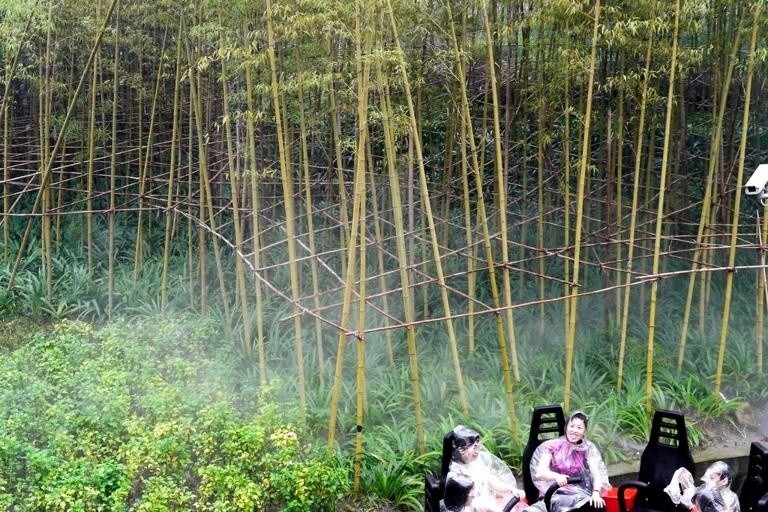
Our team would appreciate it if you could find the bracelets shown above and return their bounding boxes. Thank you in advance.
[592,490,600,493]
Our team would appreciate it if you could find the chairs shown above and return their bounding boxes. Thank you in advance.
[427,428,521,512]
[523,406,566,506]
[630,445,693,512]
[637,406,699,485]
[736,440,766,511]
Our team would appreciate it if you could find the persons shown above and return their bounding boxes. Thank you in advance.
[676,459,740,512]
[527,411,613,511]
[443,474,477,512]
[444,426,531,512]
[689,489,728,512]
